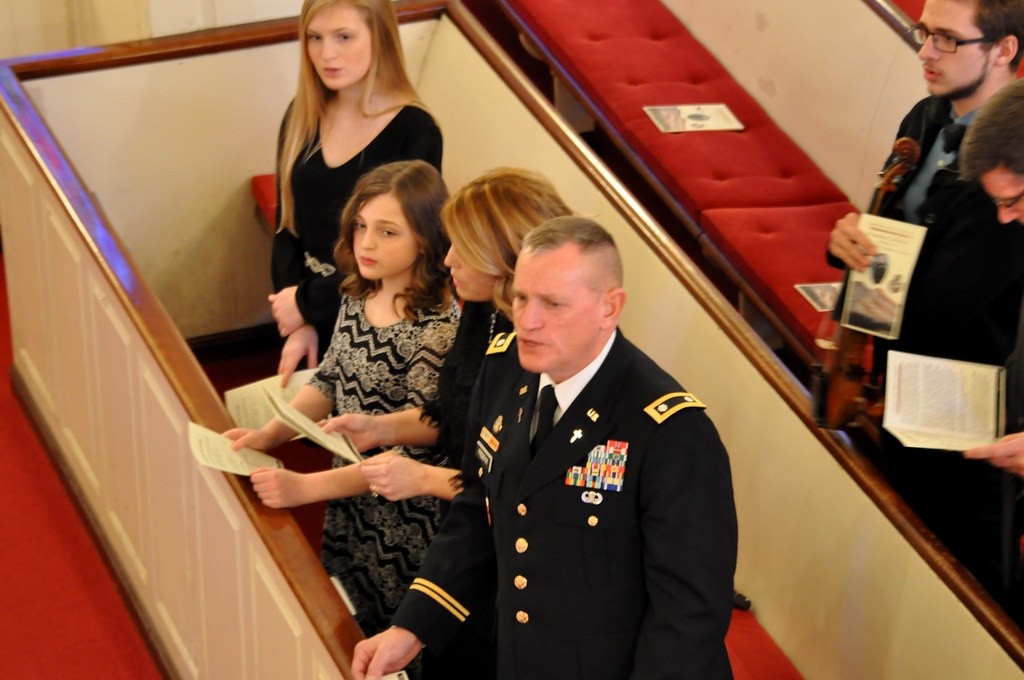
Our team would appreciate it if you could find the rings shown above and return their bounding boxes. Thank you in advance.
[371,485,378,498]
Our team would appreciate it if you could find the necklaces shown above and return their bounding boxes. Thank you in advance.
[488,307,499,344]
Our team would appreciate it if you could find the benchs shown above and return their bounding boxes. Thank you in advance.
[496,0,918,373]
[252,173,805,680]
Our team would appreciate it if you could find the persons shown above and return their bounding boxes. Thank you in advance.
[824,0,1024,610]
[219,1,739,680]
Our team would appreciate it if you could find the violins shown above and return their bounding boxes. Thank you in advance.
[811,135,923,432]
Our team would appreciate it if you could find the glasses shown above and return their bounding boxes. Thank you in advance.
[992,188,1024,209]
[911,23,1004,53]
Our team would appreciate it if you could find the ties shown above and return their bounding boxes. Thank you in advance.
[530,384,556,453]
